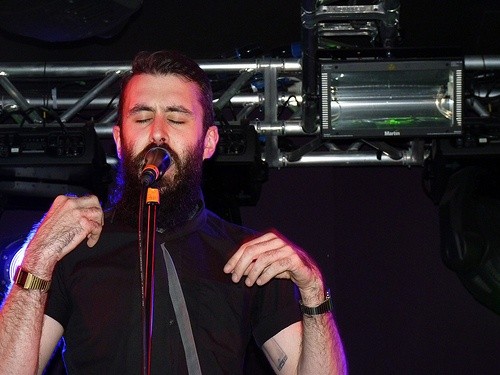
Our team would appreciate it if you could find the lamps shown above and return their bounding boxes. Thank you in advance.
[300,0,463,140]
[201,123,269,207]
[0,109,113,211]
[423,117,500,315]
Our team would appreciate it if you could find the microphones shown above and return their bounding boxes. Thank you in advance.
[139,146,172,186]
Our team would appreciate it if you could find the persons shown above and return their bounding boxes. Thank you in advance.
[0,49,347,375]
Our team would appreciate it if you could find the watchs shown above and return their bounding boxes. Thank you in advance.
[12,267,52,292]
[298,288,336,315]
